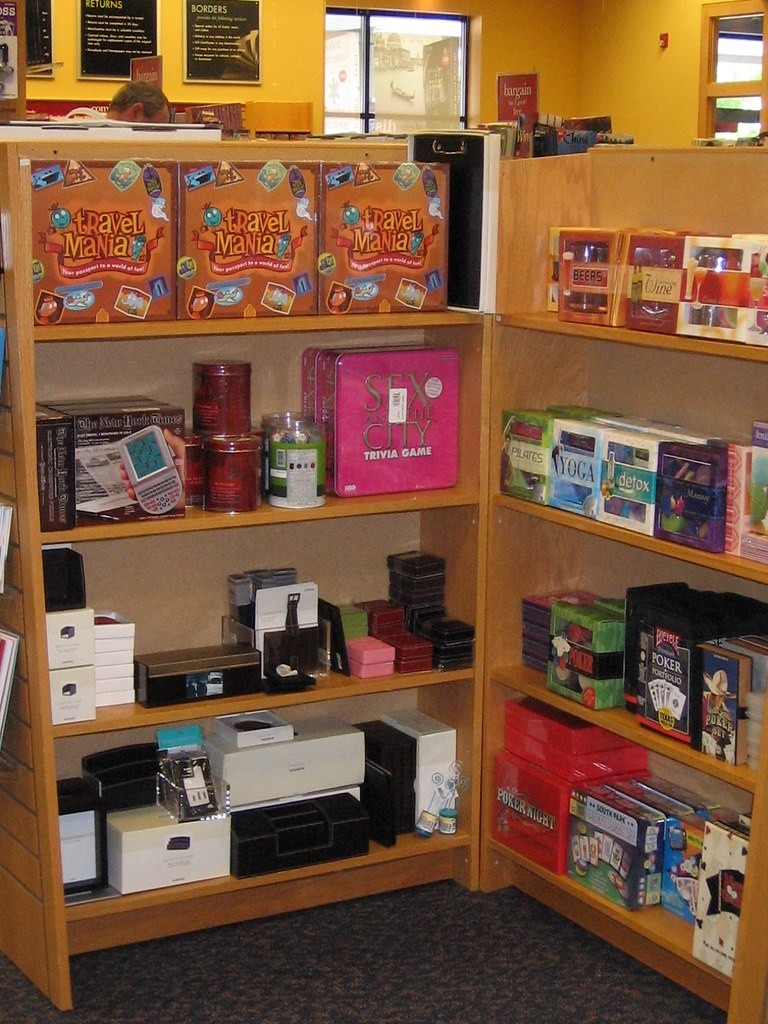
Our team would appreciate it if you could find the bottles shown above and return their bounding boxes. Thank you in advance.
[178,360,331,515]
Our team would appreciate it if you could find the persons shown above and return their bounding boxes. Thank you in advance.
[103,78,172,131]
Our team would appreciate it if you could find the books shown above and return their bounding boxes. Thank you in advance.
[476,109,768,161]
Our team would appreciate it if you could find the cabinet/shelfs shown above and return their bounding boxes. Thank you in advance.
[479,149,768,1024]
[1,140,490,1012]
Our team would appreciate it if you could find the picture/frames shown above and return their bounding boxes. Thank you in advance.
[76,0,160,81]
[182,0,261,85]
[25,0,53,78]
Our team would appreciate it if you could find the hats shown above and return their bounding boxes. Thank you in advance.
[702,670,736,700]
